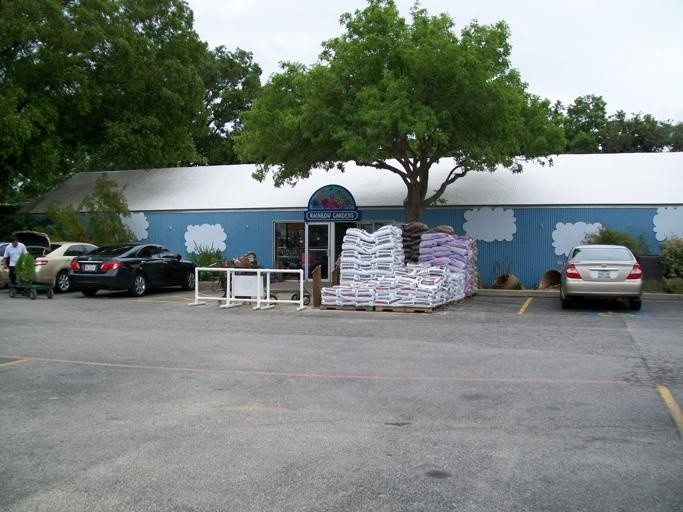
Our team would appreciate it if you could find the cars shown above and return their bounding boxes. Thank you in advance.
[0,230,99,291]
[557,245,644,311]
[68,244,200,297]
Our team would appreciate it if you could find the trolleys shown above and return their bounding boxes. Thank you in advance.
[8,284,54,299]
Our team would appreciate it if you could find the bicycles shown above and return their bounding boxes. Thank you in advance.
[291,286,311,305]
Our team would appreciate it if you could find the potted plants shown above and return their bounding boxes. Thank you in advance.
[13,252,36,286]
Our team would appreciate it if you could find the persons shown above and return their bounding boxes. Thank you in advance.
[3,236,29,294]
